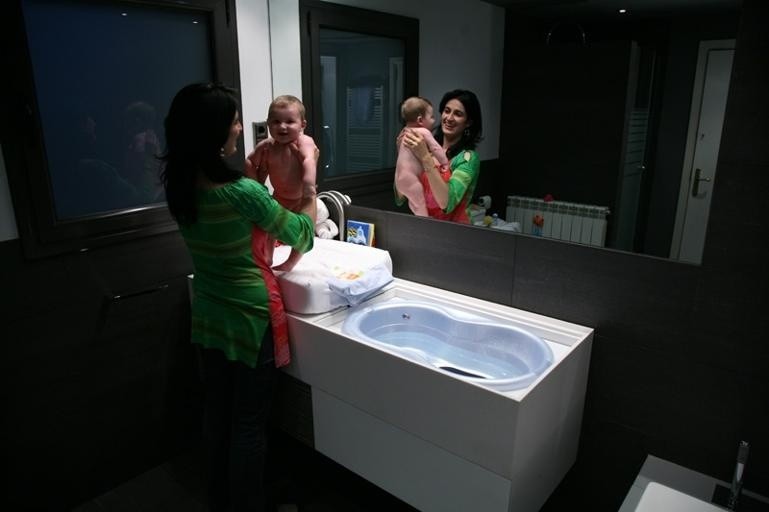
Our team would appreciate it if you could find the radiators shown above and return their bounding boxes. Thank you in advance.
[505,195,611,249]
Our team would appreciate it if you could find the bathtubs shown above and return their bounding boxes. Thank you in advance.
[343,295,554,389]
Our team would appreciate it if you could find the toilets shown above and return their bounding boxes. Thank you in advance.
[634,480,727,512]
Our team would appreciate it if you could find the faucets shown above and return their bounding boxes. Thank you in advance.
[318,190,347,239]
[725,441,748,507]
[329,188,350,208]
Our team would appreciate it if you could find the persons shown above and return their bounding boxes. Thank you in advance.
[247,96,318,272]
[392,88,485,228]
[65,96,162,212]
[122,101,163,183]
[152,81,320,512]
[395,96,450,218]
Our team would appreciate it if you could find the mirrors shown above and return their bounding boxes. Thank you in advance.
[297,0,744,269]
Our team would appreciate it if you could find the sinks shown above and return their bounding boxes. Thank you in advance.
[268,236,393,314]
